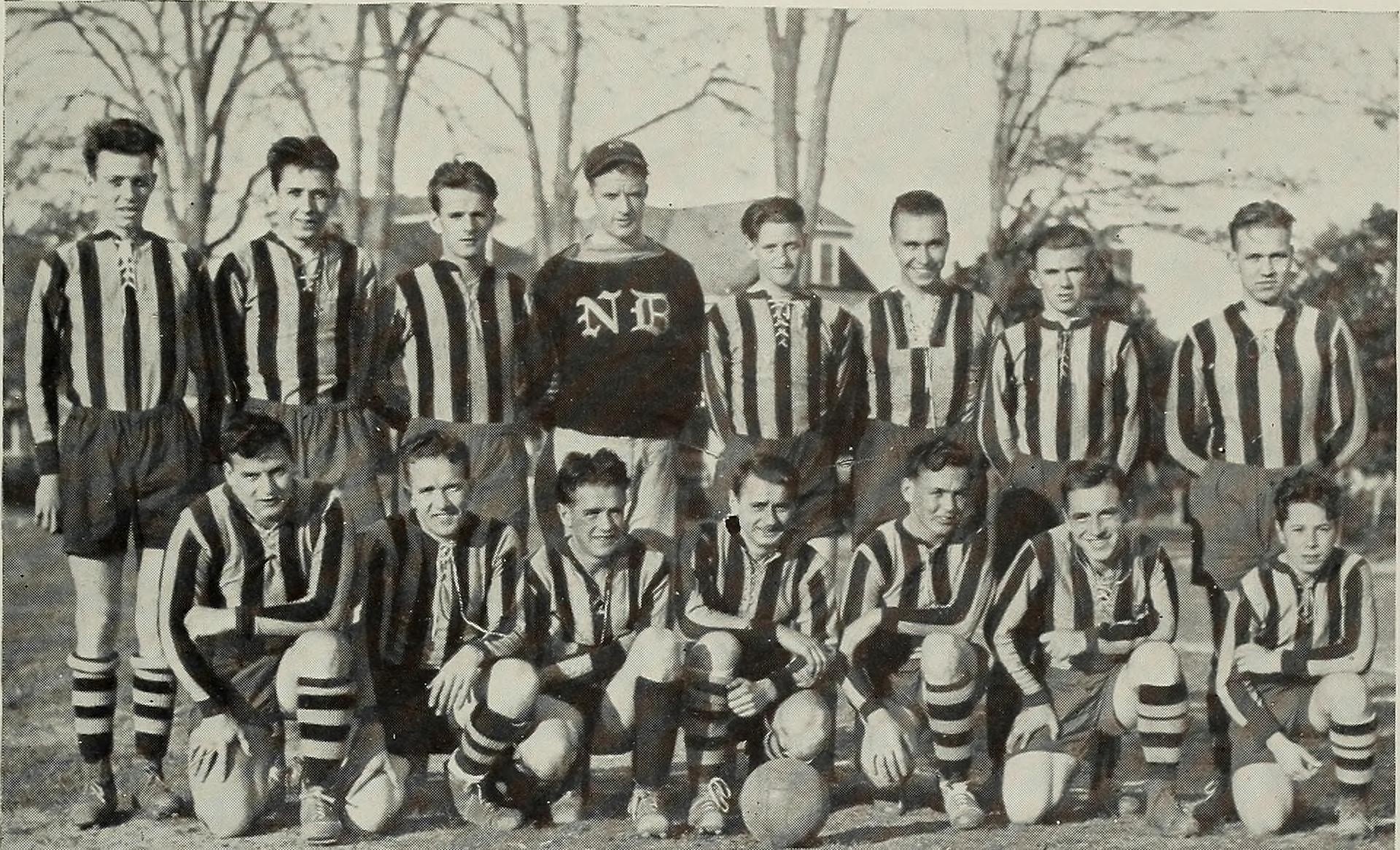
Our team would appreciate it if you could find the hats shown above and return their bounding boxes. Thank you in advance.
[584,138,649,183]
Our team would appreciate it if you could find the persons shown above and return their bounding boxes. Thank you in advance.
[153,410,364,849]
[836,434,1001,831]
[365,156,549,552]
[974,221,1148,819]
[344,427,542,835]
[669,448,844,835]
[18,117,235,828]
[523,136,708,630]
[848,190,1006,557]
[212,135,387,609]
[1159,196,1371,824]
[981,457,1204,838]
[697,194,864,582]
[507,447,691,840]
[1212,464,1378,840]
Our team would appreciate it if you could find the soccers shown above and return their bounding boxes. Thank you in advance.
[739,756,831,848]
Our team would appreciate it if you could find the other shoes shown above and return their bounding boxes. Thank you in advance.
[1088,733,1119,815]
[1192,777,1238,824]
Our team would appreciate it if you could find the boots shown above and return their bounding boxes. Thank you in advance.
[441,760,523,832]
[1145,777,1201,838]
[71,754,119,828]
[130,754,186,821]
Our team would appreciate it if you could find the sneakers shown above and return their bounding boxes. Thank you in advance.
[299,775,342,845]
[688,778,727,835]
[267,721,287,807]
[534,741,593,825]
[873,788,907,817]
[629,782,671,838]
[936,770,985,829]
[1337,786,1373,838]
